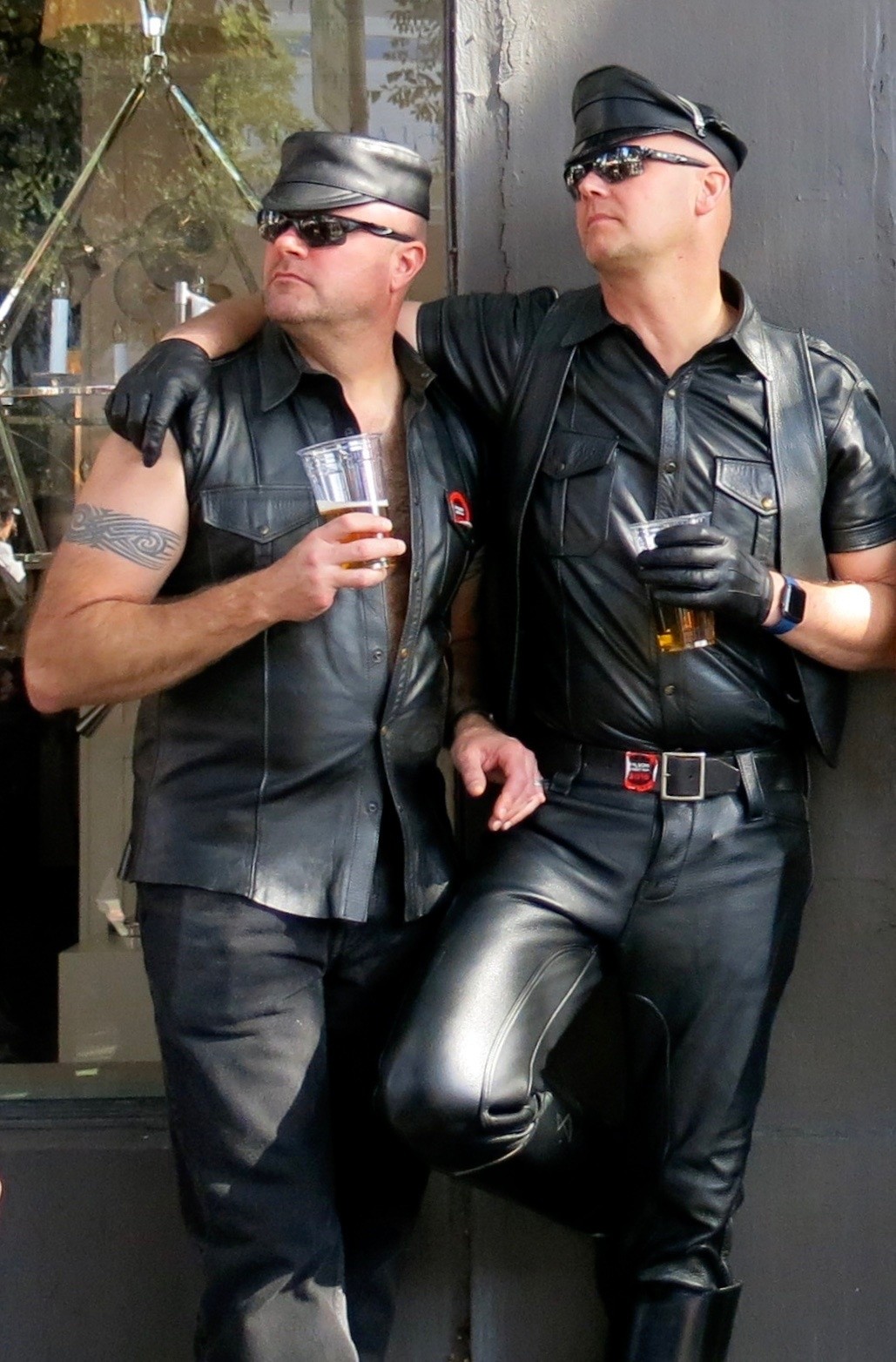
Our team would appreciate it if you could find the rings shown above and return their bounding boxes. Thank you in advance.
[534,782,543,787]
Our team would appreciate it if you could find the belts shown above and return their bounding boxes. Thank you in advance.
[516,699,804,800]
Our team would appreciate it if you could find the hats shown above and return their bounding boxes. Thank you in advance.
[260,131,434,221]
[565,65,749,188]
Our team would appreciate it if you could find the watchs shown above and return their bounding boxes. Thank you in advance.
[762,572,807,634]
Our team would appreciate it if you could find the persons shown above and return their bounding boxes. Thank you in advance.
[0,490,27,686]
[21,129,547,1362]
[103,66,896,1362]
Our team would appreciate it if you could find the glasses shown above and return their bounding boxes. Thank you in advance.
[563,147,711,201]
[257,205,415,248]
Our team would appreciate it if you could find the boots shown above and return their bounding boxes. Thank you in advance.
[450,1089,623,1305]
[606,1279,744,1362]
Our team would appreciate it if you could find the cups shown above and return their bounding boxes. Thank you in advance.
[296,434,397,578]
[629,512,718,653]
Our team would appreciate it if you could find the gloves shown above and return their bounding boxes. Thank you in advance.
[634,525,773,627]
[104,338,215,468]
